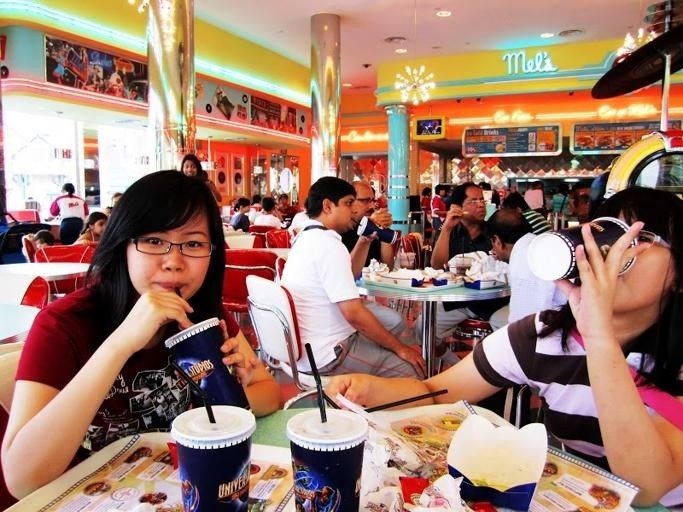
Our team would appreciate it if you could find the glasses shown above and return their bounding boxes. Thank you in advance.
[354,197,378,204]
[633,228,675,253]
[126,236,218,259]
[463,198,489,207]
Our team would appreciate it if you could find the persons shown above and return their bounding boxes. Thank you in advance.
[50,49,67,84]
[428,120,440,134]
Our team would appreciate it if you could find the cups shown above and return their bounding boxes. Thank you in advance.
[357,216,398,245]
[528,216,639,281]
[171,405,257,512]
[284,408,368,512]
[164,318,253,414]
[455,256,473,277]
[400,253,416,268]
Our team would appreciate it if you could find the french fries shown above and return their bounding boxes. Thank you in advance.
[468,475,511,493]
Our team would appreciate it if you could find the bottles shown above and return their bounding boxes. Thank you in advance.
[615,26,659,63]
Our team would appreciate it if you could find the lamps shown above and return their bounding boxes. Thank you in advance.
[616,0,657,62]
[395,0,435,106]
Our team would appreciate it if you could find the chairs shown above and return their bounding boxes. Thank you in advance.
[0,209,542,436]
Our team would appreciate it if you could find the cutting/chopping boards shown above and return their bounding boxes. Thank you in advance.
[365,276,464,292]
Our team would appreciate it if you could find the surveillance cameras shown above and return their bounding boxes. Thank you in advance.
[362,64,372,68]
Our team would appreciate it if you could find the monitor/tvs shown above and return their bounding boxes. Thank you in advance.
[412,115,445,140]
